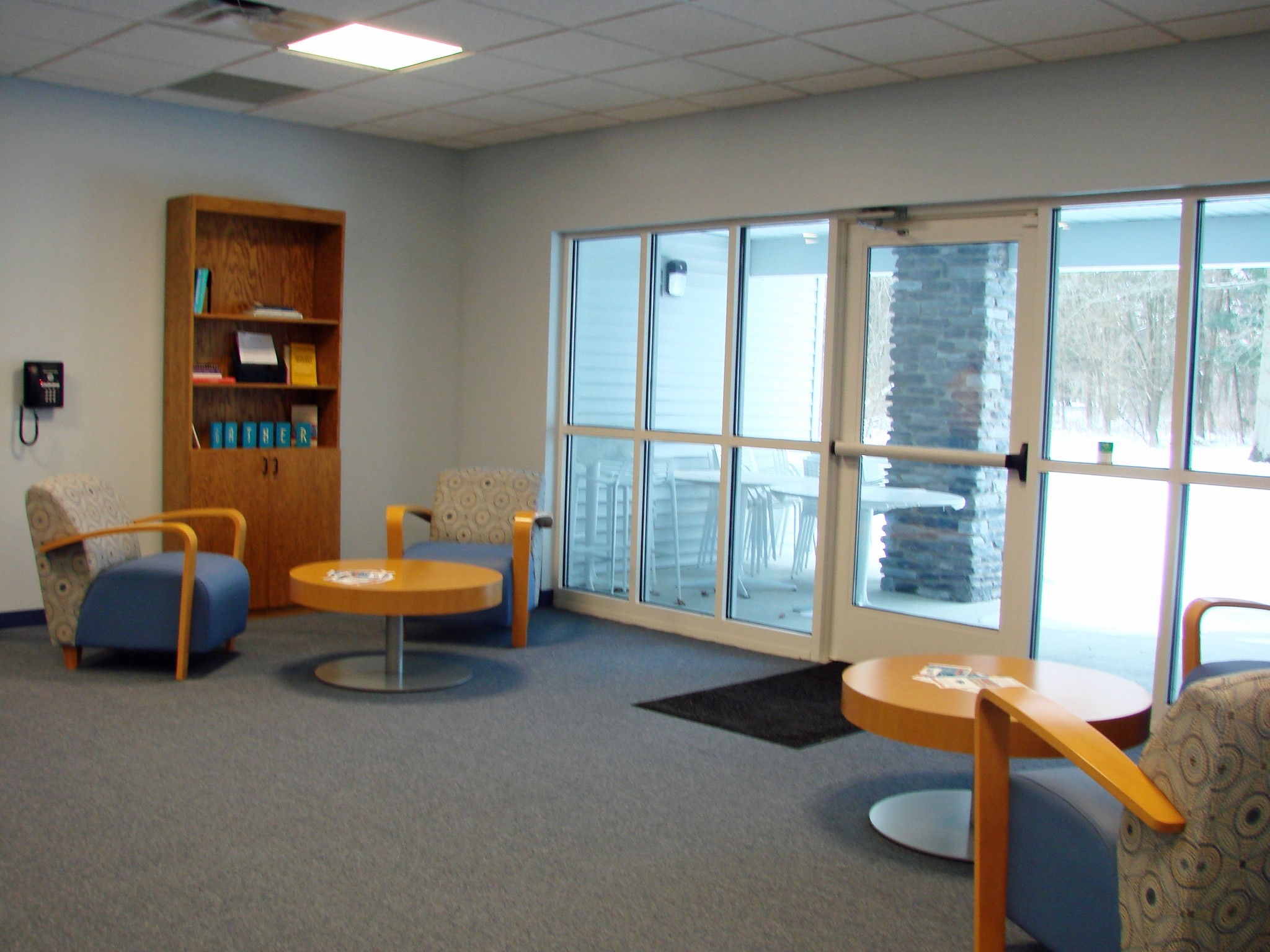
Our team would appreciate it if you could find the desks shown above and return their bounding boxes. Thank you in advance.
[831,650,1152,870]
[285,555,506,693]
[673,467,798,597]
[773,478,968,613]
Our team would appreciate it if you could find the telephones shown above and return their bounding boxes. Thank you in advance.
[24,359,63,409]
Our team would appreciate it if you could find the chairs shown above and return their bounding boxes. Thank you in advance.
[968,594,1270,952]
[573,435,885,615]
[381,462,559,656]
[24,465,255,682]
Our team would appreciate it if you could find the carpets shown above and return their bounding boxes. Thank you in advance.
[630,658,866,751]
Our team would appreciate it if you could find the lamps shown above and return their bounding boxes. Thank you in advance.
[664,257,689,299]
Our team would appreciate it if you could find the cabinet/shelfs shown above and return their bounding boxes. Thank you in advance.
[160,193,345,620]
